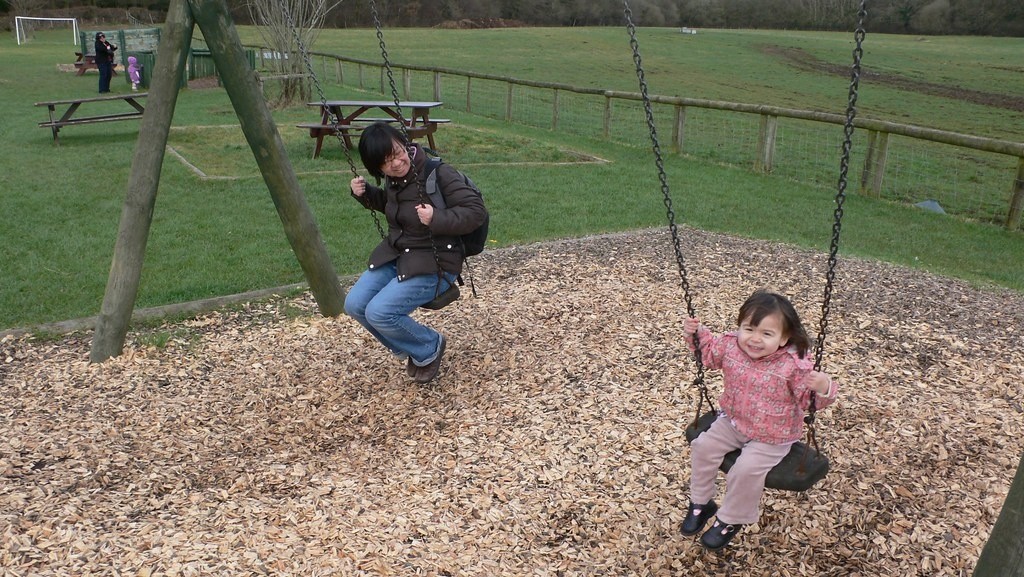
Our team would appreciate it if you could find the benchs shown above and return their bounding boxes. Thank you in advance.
[38,112,143,127]
[296,125,426,138]
[329,117,450,132]
[74,62,118,69]
[75,52,83,56]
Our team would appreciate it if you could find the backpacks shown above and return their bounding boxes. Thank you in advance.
[424,157,489,258]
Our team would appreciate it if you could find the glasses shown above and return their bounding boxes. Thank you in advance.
[381,144,407,169]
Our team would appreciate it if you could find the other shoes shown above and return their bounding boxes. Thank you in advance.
[415,335,446,383]
[407,356,417,377]
[700,516,742,550]
[680,500,717,536]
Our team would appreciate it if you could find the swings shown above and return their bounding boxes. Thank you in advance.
[279,0,461,311]
[621,0,870,492]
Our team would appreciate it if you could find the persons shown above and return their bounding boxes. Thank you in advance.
[94,32,118,93]
[127,56,144,92]
[680,291,838,553]
[343,122,488,384]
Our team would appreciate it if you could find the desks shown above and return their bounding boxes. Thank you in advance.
[77,54,117,76]
[34,93,148,146]
[308,100,443,160]
